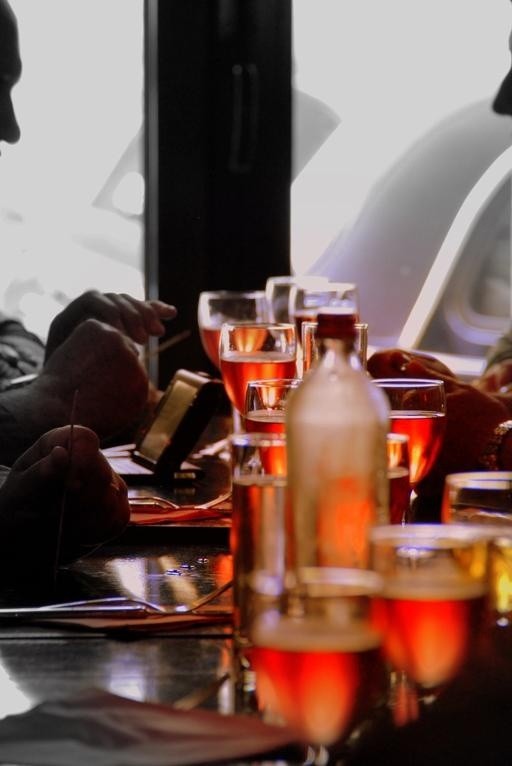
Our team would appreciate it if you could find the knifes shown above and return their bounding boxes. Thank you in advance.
[1,607,230,620]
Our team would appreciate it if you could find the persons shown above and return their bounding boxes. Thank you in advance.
[465,30,511,403]
[0,296,177,468]
[0,0,132,604]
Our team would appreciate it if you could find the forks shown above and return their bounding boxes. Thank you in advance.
[129,492,232,509]
[40,577,232,613]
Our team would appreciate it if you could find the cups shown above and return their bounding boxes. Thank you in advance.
[195,275,510,745]
